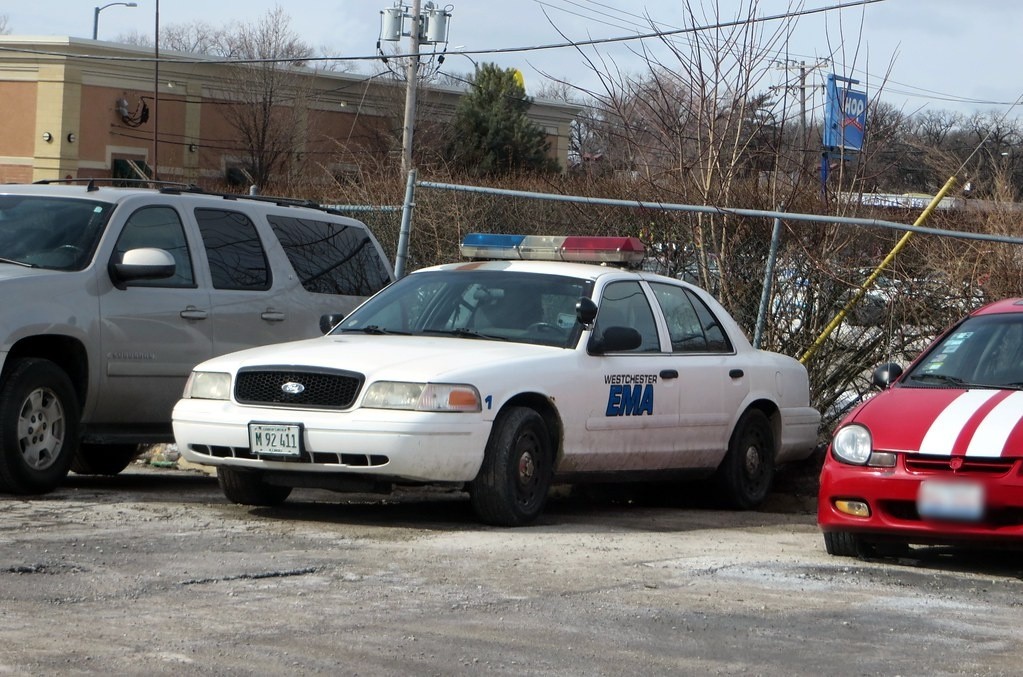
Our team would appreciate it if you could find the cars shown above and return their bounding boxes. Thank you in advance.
[818,297,1023,575]
[169,232,821,530]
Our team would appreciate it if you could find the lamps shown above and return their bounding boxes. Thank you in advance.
[42,132,52,141]
[68,133,75,142]
[297,153,304,161]
[189,144,197,152]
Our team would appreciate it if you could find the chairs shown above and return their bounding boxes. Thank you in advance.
[566,305,625,340]
[474,280,544,333]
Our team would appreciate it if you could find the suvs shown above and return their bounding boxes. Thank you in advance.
[1,175,411,497]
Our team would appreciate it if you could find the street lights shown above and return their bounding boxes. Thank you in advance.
[93,3,140,40]
